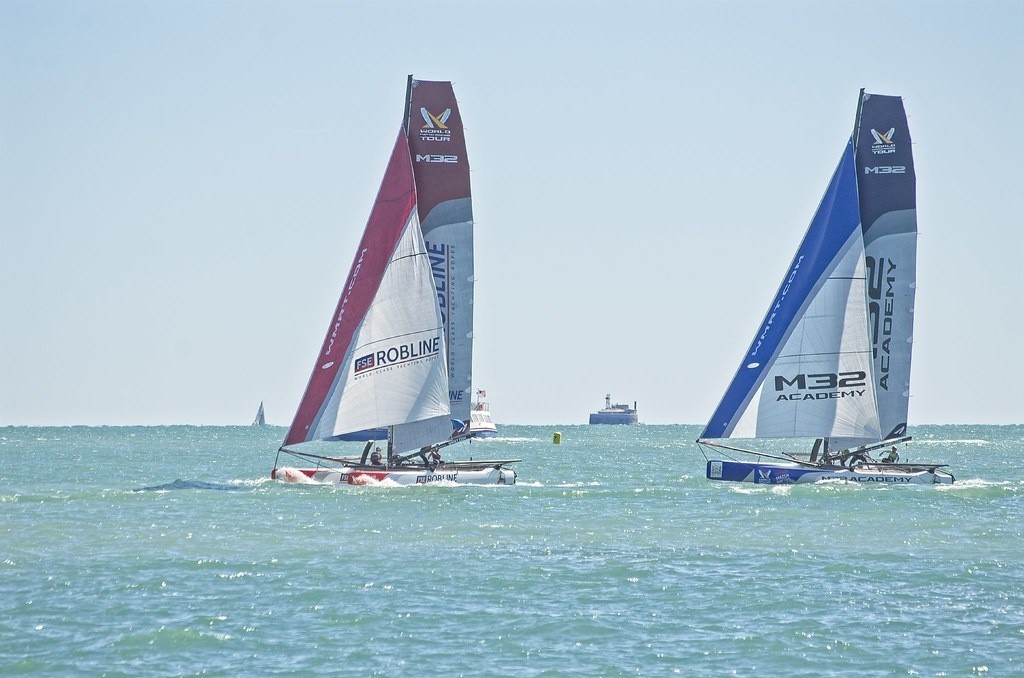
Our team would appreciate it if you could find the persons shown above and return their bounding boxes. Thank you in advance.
[849,446,874,470]
[370,447,383,465]
[428,448,445,464]
[879,447,900,464]
[818,449,850,466]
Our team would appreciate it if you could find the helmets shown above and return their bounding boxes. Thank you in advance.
[376,447,381,451]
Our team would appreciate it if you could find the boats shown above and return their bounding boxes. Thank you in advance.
[589,393,639,425]
[322,387,498,441]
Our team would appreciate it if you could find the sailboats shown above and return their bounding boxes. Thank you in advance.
[271,75,522,488]
[696,85,956,487]
[251,401,265,426]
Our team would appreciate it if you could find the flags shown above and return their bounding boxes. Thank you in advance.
[479,390,486,397]
